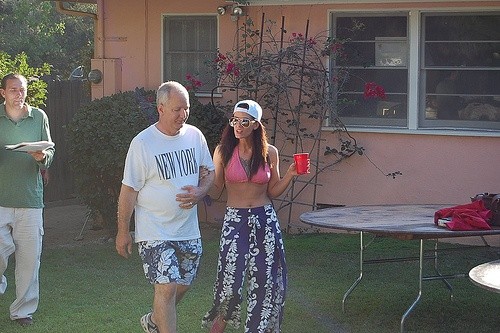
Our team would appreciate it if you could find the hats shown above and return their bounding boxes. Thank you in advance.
[233,99,262,122]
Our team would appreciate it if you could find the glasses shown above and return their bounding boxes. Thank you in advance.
[228,117,257,128]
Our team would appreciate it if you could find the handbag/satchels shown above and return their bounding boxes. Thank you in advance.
[433,200,493,231]
[469,193,500,227]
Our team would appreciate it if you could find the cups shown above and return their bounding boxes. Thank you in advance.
[294,153,309,174]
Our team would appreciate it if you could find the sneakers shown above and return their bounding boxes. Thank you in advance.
[140,313,160,333]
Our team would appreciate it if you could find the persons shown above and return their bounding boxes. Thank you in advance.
[0,73,55,326]
[436,71,469,120]
[116,81,215,333]
[200,99,310,333]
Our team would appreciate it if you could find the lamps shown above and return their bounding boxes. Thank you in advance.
[217,3,244,15]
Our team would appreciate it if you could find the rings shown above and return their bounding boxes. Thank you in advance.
[189,201,193,205]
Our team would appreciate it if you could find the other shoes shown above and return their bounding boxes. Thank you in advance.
[16,317,32,326]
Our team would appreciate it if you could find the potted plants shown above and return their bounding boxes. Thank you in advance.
[62,86,232,232]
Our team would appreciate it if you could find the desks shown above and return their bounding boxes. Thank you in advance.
[299,204,500,333]
